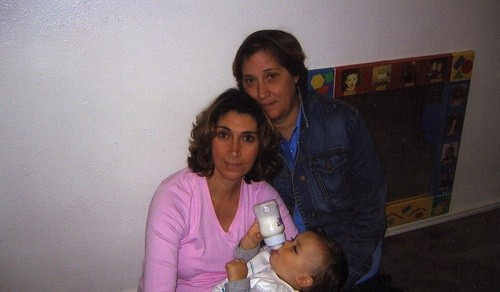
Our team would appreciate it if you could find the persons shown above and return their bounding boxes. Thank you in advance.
[135,88,299,292]
[232,30,386,292]
[212,214,349,292]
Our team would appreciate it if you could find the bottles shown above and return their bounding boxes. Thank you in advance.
[253,199,286,251]
